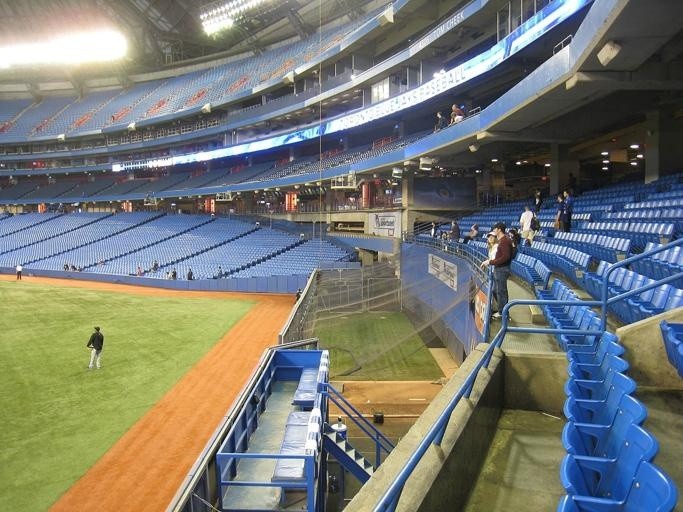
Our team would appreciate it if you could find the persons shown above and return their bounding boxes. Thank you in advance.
[15,263,21,280]
[533,186,543,212]
[451,104,465,118]
[171,267,176,280]
[152,260,158,271]
[479,206,541,323]
[429,220,436,238]
[562,190,573,216]
[449,219,460,242]
[553,193,570,232]
[187,268,192,280]
[569,172,578,197]
[447,112,463,126]
[135,266,143,276]
[216,266,222,278]
[433,111,448,132]
[86,326,104,369]
[462,223,478,244]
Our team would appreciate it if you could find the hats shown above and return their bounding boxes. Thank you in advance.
[491,221,506,230]
[487,231,497,237]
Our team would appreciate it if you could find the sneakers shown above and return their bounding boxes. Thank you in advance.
[491,309,510,320]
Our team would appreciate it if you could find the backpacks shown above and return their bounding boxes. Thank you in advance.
[530,211,540,232]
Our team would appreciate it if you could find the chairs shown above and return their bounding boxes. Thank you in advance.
[417,174,682,512]
[0,0,435,280]
